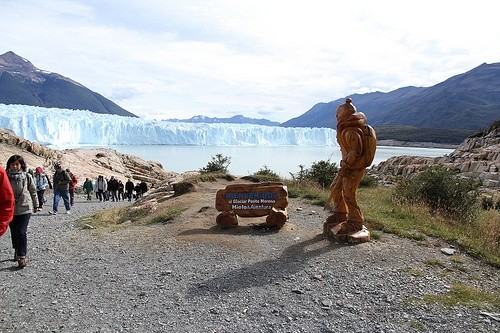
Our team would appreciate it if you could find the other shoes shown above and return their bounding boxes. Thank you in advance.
[47,210,57,215]
[64,208,70,215]
[12,249,27,269]
[37,208,43,211]
[71,203,74,206]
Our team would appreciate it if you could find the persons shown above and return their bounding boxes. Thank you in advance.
[326,98,376,231]
[0,166,14,236]
[5,155,39,267]
[28,164,160,215]
